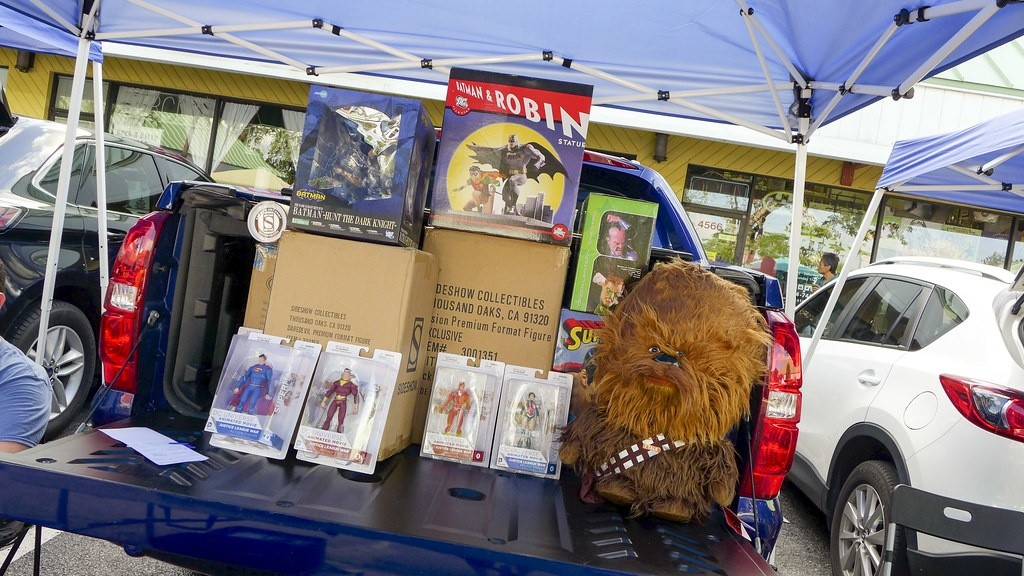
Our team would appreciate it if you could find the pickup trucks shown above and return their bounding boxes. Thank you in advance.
[0,143,806,576]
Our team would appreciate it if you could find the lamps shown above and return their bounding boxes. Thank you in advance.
[15,50,35,73]
[653,132,670,163]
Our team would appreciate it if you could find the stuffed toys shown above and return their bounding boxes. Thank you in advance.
[553,256,775,526]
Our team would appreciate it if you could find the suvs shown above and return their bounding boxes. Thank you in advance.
[0,114,218,442]
[782,254,1023,576]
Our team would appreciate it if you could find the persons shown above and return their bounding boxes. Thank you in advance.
[812,253,840,293]
[0,259,52,455]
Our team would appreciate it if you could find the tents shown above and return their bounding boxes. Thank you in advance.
[0,0,1024,368]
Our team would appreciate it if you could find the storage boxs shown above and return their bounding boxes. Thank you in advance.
[264,230,441,463]
[240,244,277,330]
[408,228,570,446]
[430,67,594,246]
[287,84,438,250]
[553,309,621,374]
[567,193,661,314]
